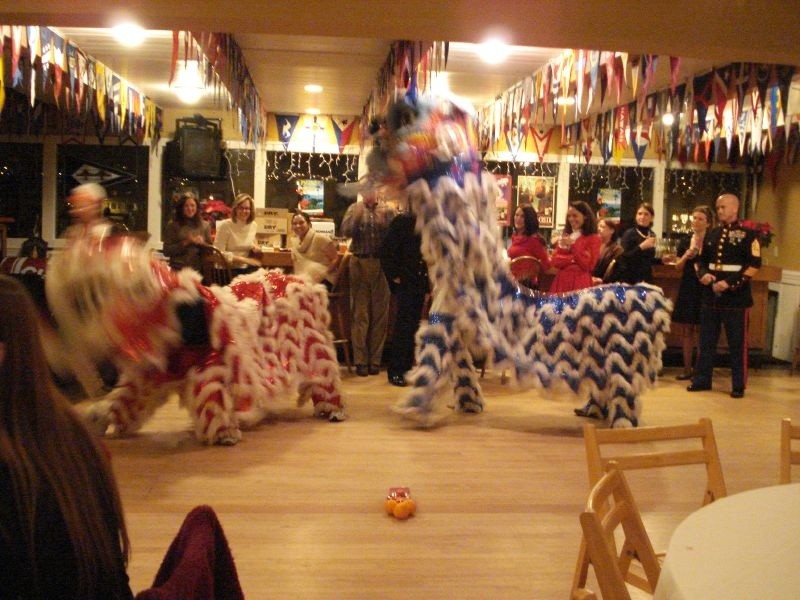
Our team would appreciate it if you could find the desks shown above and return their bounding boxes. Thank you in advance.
[652,485,800,600]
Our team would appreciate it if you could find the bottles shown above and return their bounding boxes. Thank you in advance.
[657,231,687,258]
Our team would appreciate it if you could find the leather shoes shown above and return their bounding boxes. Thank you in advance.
[388,376,408,387]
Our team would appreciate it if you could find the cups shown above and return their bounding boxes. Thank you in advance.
[273,241,280,251]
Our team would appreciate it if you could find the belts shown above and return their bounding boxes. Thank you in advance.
[354,253,380,259]
[708,263,742,272]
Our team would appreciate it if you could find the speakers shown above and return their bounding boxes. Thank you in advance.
[176,127,222,181]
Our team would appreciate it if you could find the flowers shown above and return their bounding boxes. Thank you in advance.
[740,219,777,248]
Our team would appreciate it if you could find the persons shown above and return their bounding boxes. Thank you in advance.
[213,192,259,286]
[592,217,623,277]
[615,202,657,286]
[507,203,550,289]
[60,184,131,388]
[687,194,761,397]
[379,191,433,386]
[548,200,601,294]
[292,212,339,292]
[670,206,712,379]
[164,193,210,275]
[343,175,394,376]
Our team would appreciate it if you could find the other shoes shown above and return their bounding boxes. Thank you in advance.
[368,365,379,374]
[686,384,712,392]
[356,366,368,376]
[730,391,745,399]
[675,371,694,380]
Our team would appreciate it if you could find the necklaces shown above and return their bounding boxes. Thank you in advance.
[636,228,651,239]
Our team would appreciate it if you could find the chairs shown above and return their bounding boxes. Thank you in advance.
[481,255,543,376]
[326,253,352,372]
[579,459,662,600]
[780,417,800,483]
[134,505,247,600]
[570,416,726,600]
